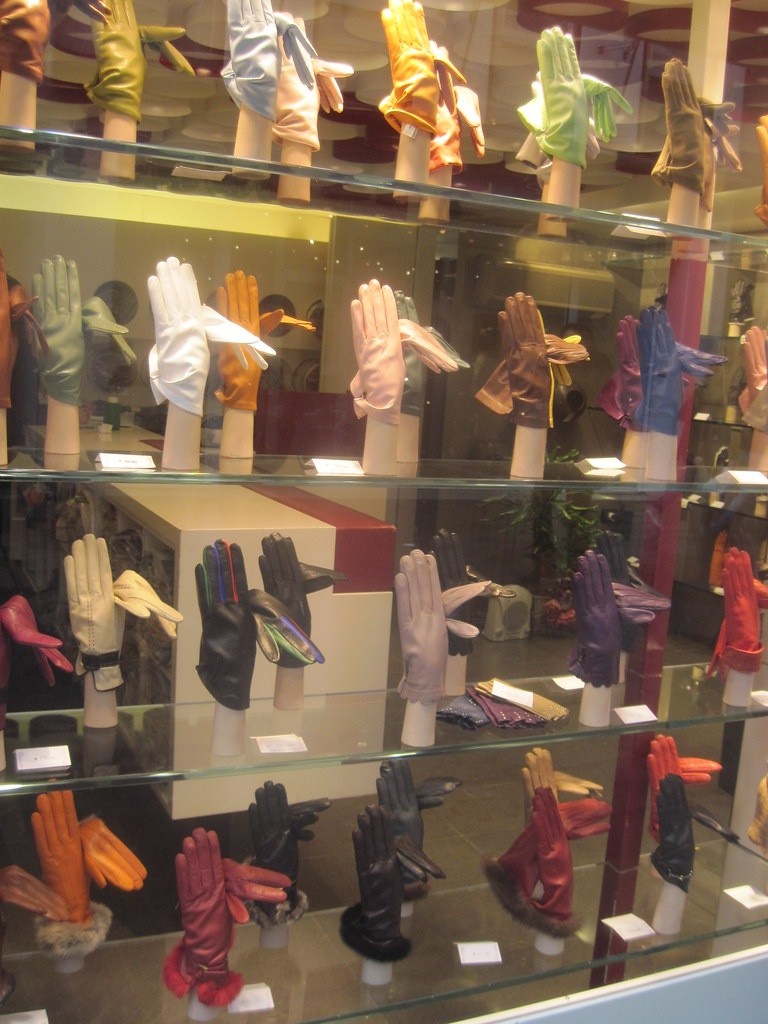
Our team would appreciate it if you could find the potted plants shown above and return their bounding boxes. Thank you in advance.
[484,441,601,633]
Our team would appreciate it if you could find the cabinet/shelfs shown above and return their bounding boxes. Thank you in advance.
[640,255,768,643]
[0,122,768,1024]
[19,423,395,821]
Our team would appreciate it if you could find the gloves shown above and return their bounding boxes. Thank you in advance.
[0,733,768,1000]
[1,1,768,217]
[0,256,768,444]
[2,529,766,707]
